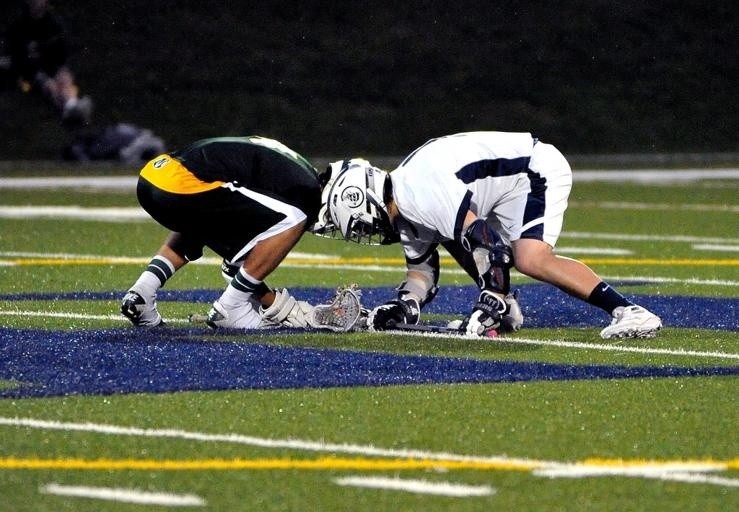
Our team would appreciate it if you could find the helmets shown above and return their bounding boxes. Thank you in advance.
[310,156,401,248]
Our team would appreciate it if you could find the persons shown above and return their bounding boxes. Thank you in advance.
[114,134,372,336]
[63,119,193,170]
[15,0,98,167]
[325,127,666,343]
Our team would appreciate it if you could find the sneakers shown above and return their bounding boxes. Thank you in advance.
[446,288,525,333]
[204,297,267,331]
[119,282,165,329]
[599,304,663,341]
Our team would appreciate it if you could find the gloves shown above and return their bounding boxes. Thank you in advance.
[258,285,313,329]
[458,290,511,336]
[366,293,422,334]
